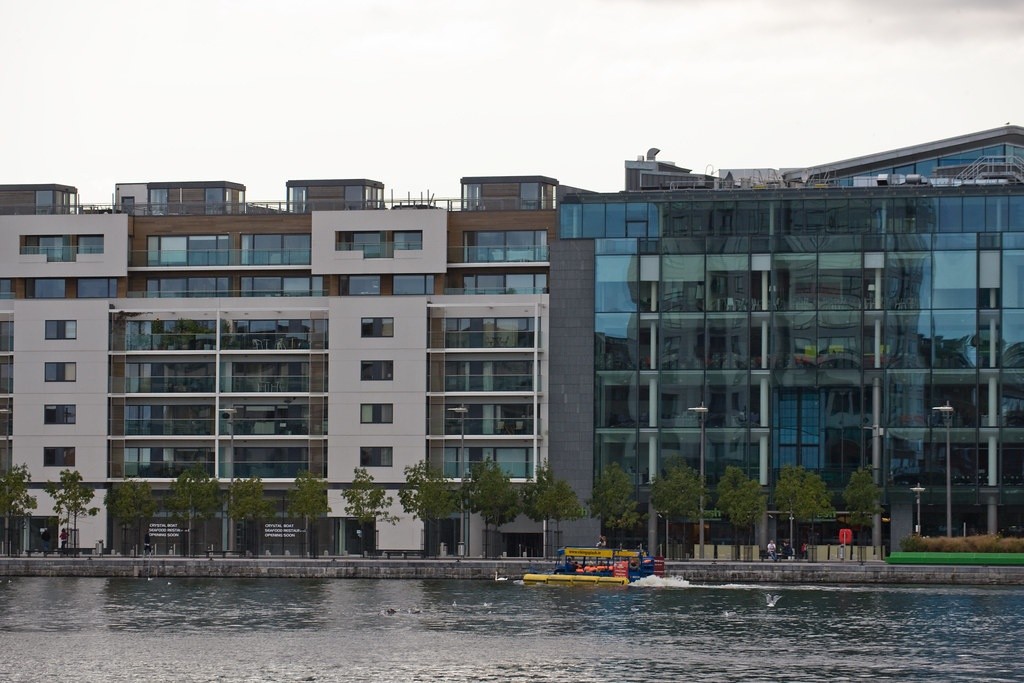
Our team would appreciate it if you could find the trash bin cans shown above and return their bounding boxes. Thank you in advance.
[837,545,846,562]
[96,540,103,558]
[457,542,465,559]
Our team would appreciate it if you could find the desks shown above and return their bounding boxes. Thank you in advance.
[263,339,271,349]
[257,382,271,392]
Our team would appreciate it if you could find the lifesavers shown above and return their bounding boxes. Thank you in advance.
[629,559,639,569]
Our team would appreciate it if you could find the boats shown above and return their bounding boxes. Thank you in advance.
[522,542,667,588]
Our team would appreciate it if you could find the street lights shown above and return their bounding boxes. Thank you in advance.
[931,400,952,538]
[687,401,708,559]
[910,482,926,536]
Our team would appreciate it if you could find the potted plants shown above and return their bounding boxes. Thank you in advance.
[150,318,233,350]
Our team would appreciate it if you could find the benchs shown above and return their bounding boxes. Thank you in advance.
[204,551,244,558]
[25,550,67,558]
[758,554,801,562]
[384,551,427,560]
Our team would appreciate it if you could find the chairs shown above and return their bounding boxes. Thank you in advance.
[252,339,263,349]
[272,382,280,392]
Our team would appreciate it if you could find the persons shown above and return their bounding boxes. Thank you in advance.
[567,556,582,571]
[59,528,69,555]
[41,530,51,553]
[143,530,152,555]
[766,540,808,561]
